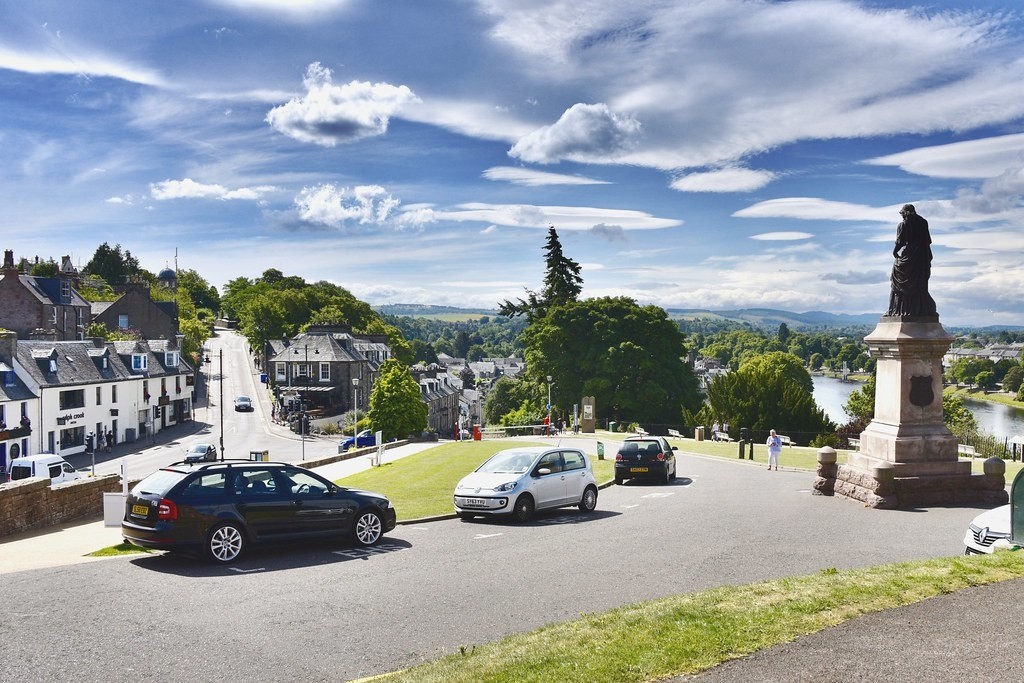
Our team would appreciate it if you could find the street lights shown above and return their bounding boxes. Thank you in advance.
[351,378,359,449]
[546,375,553,435]
[203,348,224,460]
[462,410,466,440]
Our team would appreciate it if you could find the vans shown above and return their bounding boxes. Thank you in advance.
[6,453,79,486]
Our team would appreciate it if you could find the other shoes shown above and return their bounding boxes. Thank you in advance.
[767,467,771,470]
[774,467,778,471]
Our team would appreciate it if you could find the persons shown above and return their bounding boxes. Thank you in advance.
[270,403,293,427]
[105,430,114,454]
[723,420,730,443]
[766,429,782,471]
[541,415,551,436]
[883,204,937,317]
[556,418,564,434]
[249,346,253,355]
[98,430,105,452]
[711,420,721,442]
[254,356,258,369]
[574,416,580,432]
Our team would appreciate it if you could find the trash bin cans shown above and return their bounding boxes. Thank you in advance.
[739,428,749,444]
[250,450,269,462]
[609,422,617,432]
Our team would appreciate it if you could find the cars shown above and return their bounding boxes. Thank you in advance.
[339,428,395,451]
[613,435,679,486]
[452,447,599,525]
[183,443,217,462]
[961,504,1012,558]
[234,396,252,412]
[119,456,397,565]
[458,429,472,440]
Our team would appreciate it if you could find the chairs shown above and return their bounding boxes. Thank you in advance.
[514,455,531,471]
[628,444,638,451]
[252,480,266,492]
[648,445,658,450]
[236,476,251,491]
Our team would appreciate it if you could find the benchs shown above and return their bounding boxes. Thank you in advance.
[668,429,684,440]
[635,427,649,436]
[957,444,982,460]
[305,406,325,419]
[478,427,506,438]
[848,438,860,452]
[367,445,385,467]
[776,435,796,448]
[715,432,734,444]
